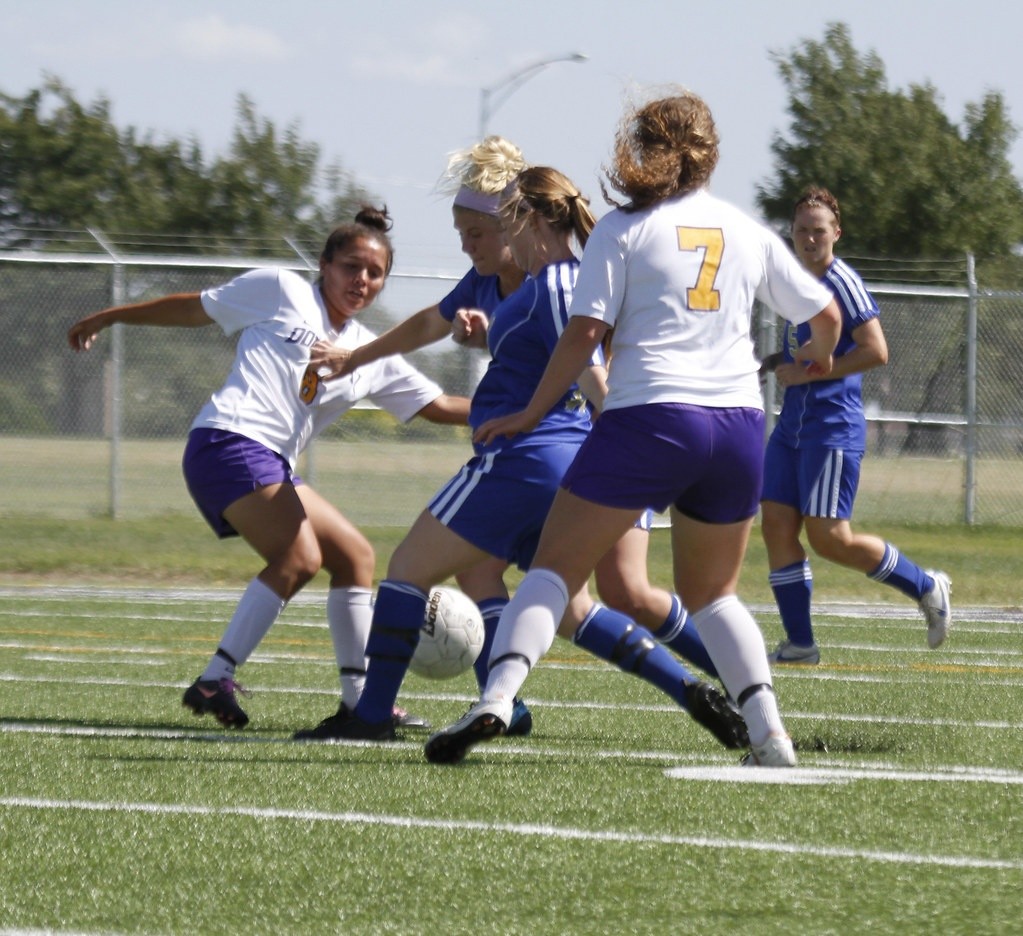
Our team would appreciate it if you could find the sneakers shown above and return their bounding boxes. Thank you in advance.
[739,732,798,769]
[290,701,397,742]
[182,674,254,730]
[424,699,515,766]
[335,699,430,728]
[507,698,532,737]
[681,676,752,750]
[766,639,820,666]
[918,567,954,648]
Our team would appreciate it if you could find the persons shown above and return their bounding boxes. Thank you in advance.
[425,94,838,764]
[68,206,470,728]
[760,185,952,662]
[292,135,752,749]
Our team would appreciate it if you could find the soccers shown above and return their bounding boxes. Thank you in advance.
[407,585,485,681]
